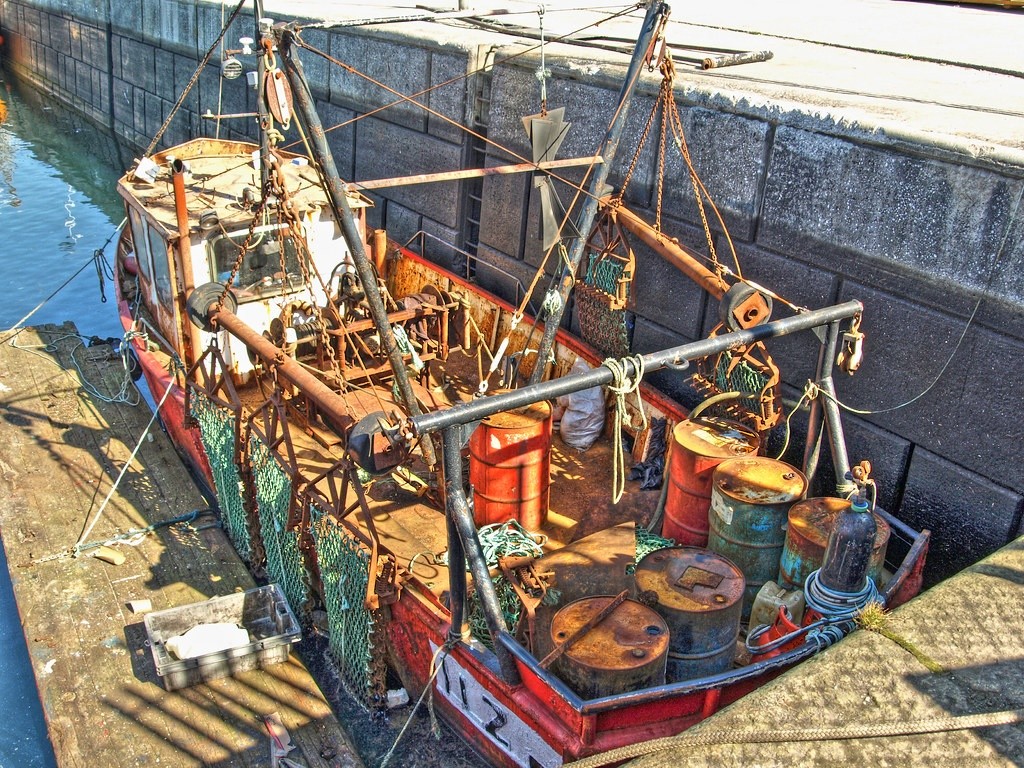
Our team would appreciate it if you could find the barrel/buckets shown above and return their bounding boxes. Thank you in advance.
[469,389,554,533]
[705,457,809,623]
[633,546,746,684]
[747,581,805,634]
[778,497,892,593]
[661,416,761,548]
[549,595,669,702]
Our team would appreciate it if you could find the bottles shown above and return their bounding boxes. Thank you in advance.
[165,623,250,660]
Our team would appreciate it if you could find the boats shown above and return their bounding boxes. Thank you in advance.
[114,0,931,768]
